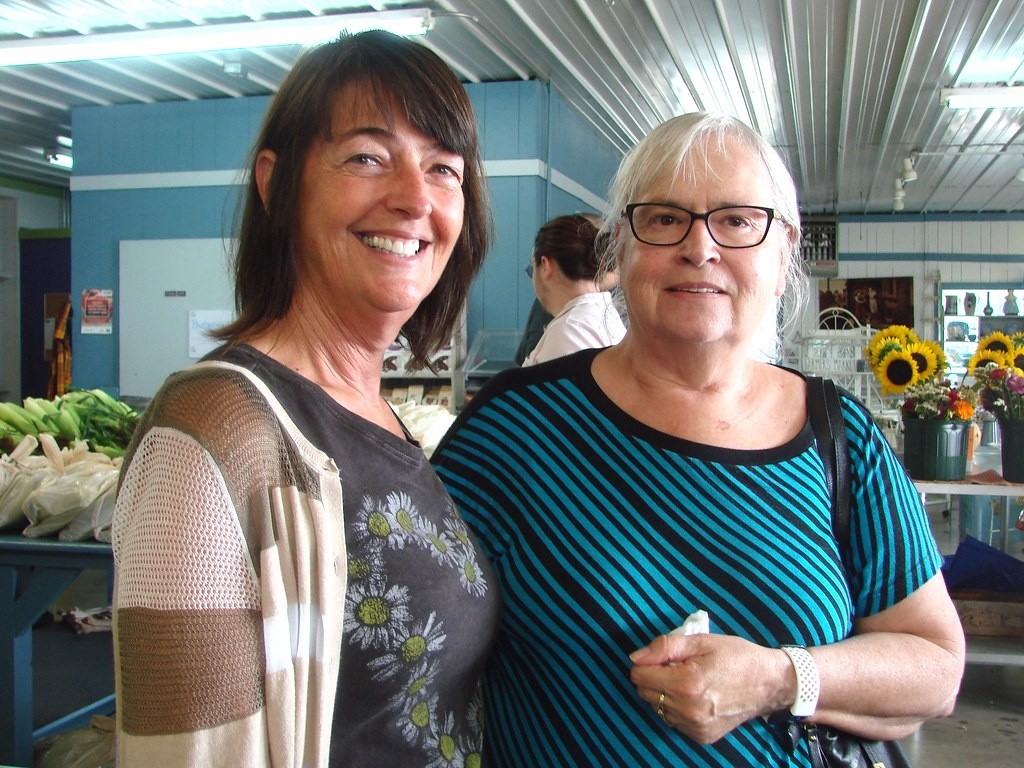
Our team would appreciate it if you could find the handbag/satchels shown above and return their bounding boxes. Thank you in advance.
[780,710,913,768]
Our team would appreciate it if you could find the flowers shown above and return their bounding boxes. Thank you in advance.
[863,324,980,421]
[968,330,1024,420]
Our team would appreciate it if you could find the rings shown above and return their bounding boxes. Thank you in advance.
[657,694,669,723]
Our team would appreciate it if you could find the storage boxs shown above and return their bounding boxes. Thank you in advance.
[951,586,1024,638]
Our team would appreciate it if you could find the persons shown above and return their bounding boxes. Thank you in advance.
[429,112,968,768]
[110,30,498,768]
[513,212,629,367]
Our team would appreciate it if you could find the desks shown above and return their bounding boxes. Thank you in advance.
[0,531,116,768]
[910,479,1024,667]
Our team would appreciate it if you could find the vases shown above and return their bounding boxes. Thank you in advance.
[997,418,1024,484]
[903,416,972,479]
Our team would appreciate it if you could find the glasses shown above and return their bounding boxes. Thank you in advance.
[525,257,541,278]
[618,201,786,249]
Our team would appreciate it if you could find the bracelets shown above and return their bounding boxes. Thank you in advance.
[762,644,819,724]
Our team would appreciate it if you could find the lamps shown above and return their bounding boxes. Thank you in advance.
[893,178,906,199]
[939,85,1024,109]
[44,147,72,169]
[0,8,436,67]
[902,152,917,182]
[893,199,905,211]
